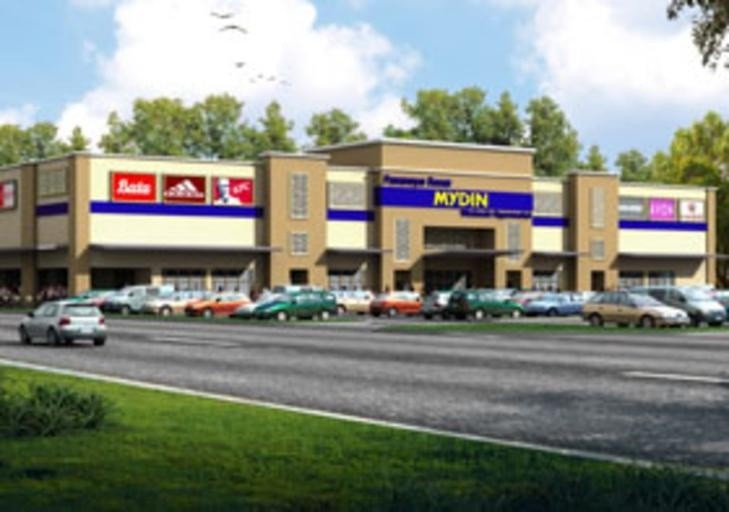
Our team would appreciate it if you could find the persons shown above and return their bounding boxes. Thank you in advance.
[214,177,242,205]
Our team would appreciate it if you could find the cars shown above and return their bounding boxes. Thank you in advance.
[582,289,691,328]
[637,284,727,327]
[80,285,338,322]
[339,281,583,320]
[19,300,108,347]
